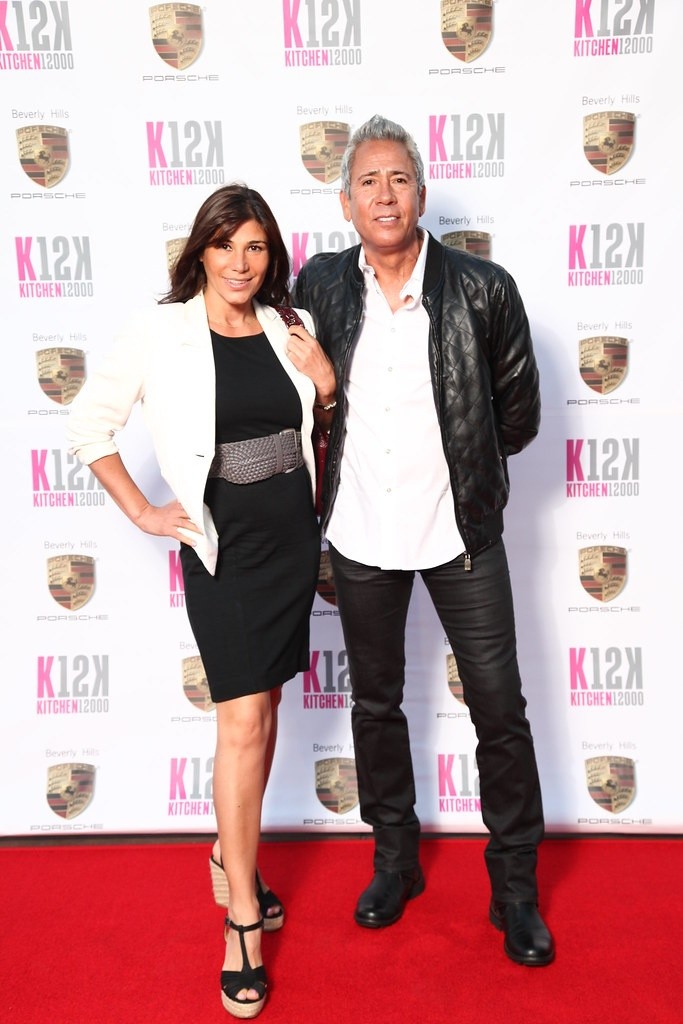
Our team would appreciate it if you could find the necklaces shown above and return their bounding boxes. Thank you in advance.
[209,317,256,327]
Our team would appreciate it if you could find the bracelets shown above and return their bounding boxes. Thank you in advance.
[315,400,338,410]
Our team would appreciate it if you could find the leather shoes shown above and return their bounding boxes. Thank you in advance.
[489,897,555,967]
[353,862,425,928]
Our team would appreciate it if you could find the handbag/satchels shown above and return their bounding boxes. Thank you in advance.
[275,305,334,515]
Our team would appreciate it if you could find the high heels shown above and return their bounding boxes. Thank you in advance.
[209,846,284,930]
[220,910,268,1018]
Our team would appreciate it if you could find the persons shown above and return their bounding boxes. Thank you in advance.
[67,185,335,1019]
[295,116,557,966]
